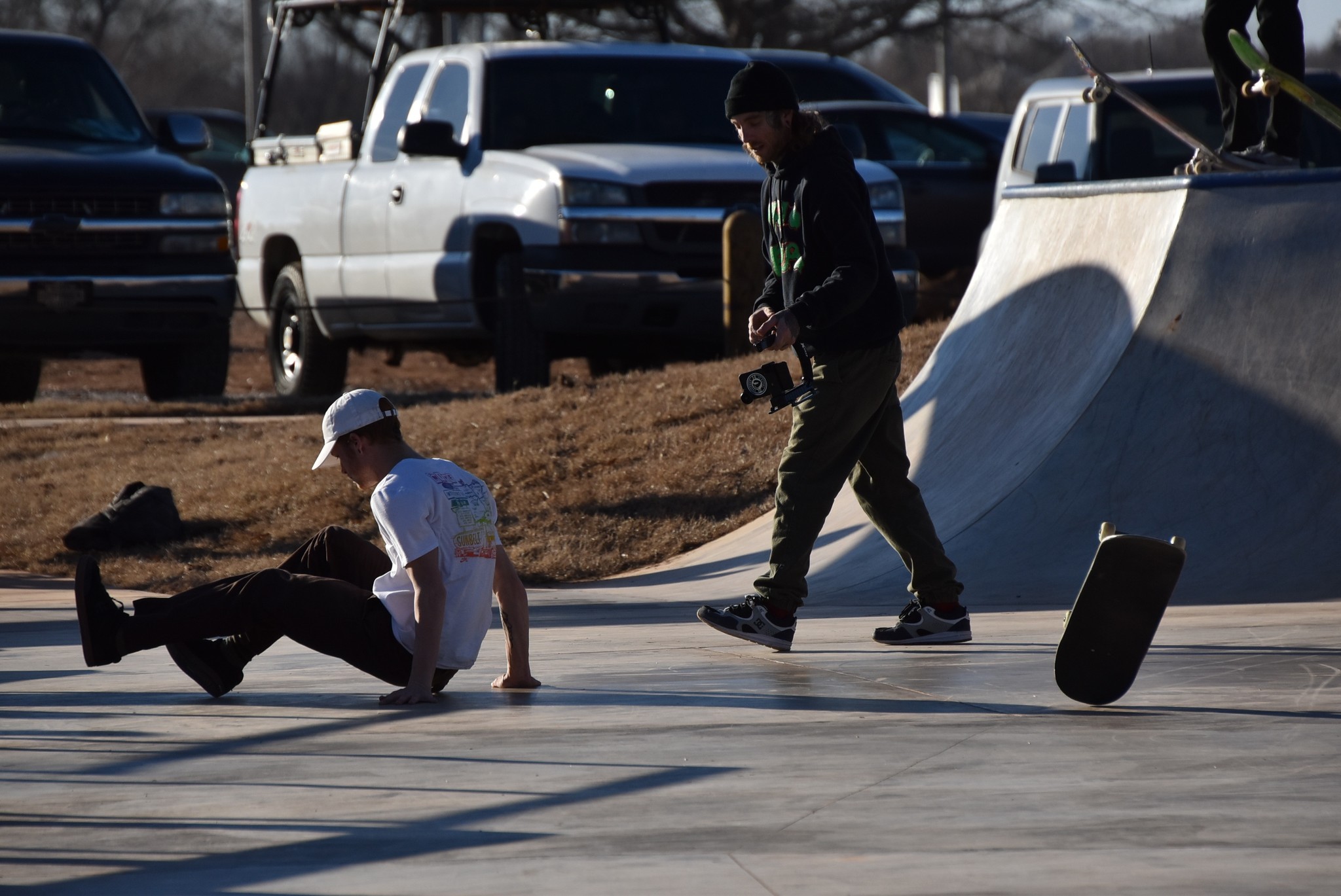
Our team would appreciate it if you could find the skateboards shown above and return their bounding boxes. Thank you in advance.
[1228,29,1341,131]
[1054,523,1190,706]
[1064,35,1244,177]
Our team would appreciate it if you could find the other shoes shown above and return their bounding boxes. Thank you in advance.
[1232,140,1306,169]
[74,552,129,667]
[1175,146,1261,175]
[60,482,183,552]
[166,638,244,698]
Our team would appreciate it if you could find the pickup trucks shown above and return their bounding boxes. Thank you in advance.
[231,40,911,417]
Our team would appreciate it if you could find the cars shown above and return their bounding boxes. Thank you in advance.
[150,105,268,192]
[734,50,1022,274]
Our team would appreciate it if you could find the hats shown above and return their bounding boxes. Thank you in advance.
[724,60,801,119]
[310,389,399,471]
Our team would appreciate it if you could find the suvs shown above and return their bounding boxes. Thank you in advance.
[0,29,241,405]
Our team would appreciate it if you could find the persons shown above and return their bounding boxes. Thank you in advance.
[697,62,973,651]
[75,388,541,704]
[1188,0,1307,165]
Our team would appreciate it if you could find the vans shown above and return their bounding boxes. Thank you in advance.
[991,62,1340,216]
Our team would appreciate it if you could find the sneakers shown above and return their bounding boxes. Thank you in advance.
[872,600,973,645]
[696,594,797,652]
